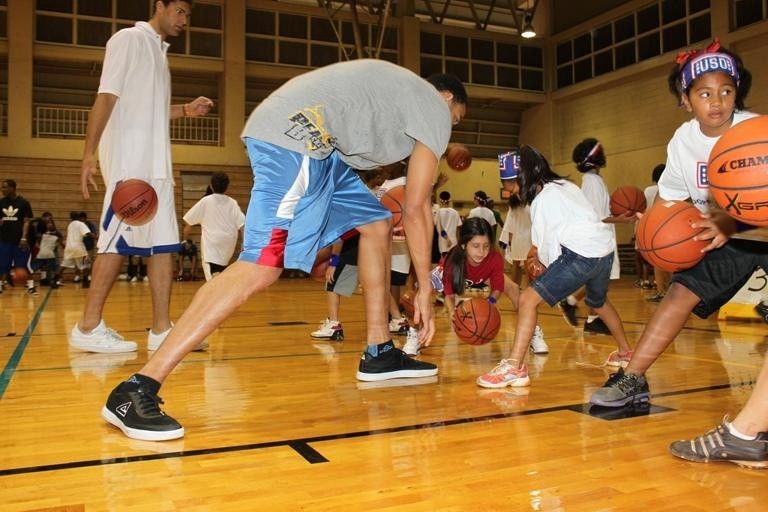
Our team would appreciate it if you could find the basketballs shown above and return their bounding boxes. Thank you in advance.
[525,248,547,282]
[610,186,644,216]
[311,262,330,283]
[380,146,477,322]
[113,180,156,225]
[705,117,768,229]
[635,200,711,272]
[454,299,499,344]
[12,268,27,286]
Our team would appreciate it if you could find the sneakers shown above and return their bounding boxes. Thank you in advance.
[69,320,209,440]
[0,273,195,295]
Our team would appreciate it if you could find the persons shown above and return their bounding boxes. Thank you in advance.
[183,103,191,117]
[100,57,467,443]
[428,191,538,282]
[183,168,246,281]
[588,35,768,409]
[401,216,549,356]
[304,160,410,337]
[557,137,637,338]
[474,146,635,389]
[1,179,95,291]
[66,0,214,358]
[670,350,768,470]
[129,239,199,283]
[643,164,666,303]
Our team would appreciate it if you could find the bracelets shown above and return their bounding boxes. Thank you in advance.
[488,295,496,304]
